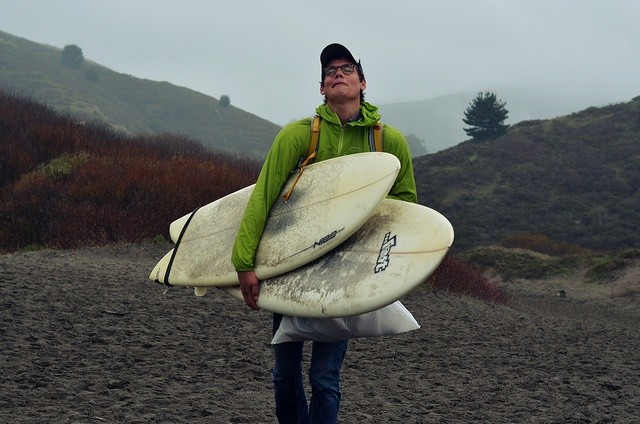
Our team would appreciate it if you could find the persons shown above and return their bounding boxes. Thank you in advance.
[232,43,418,424]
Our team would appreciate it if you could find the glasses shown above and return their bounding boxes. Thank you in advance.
[324,63,357,76]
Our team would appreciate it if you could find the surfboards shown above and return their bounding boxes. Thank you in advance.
[168,197,455,319]
[149,150,401,286]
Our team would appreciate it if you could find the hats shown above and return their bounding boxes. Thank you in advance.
[319,41,366,84]
